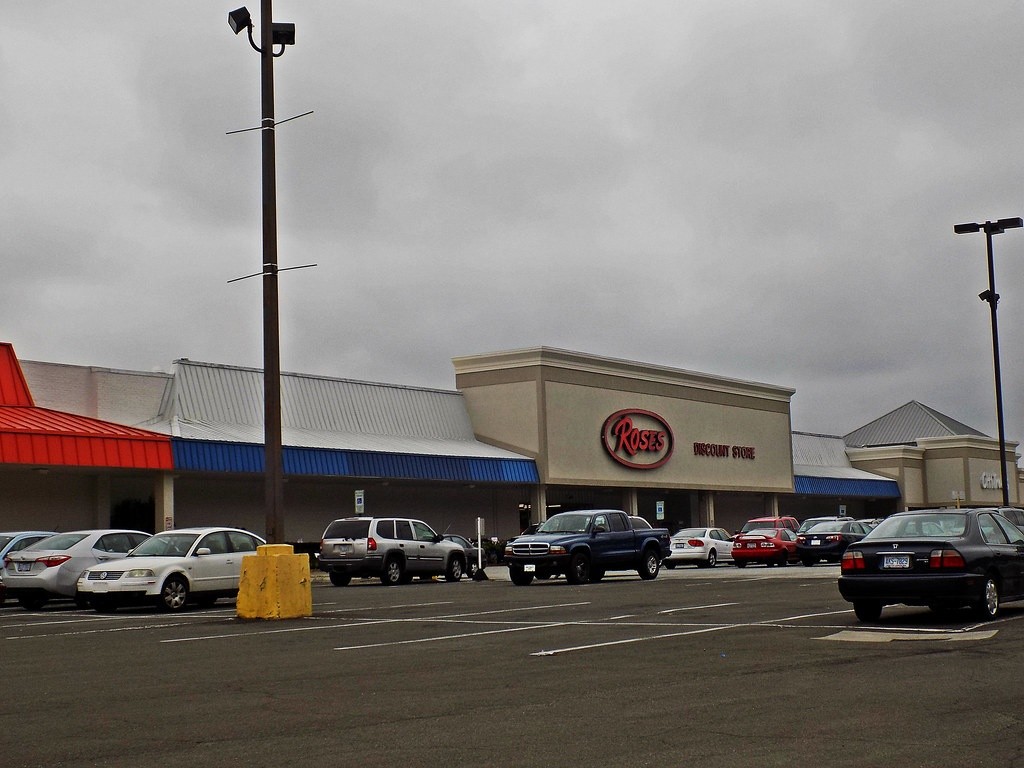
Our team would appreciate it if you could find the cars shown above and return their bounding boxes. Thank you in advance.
[0,531,60,605]
[420,534,486,579]
[796,516,854,537]
[798,521,873,567]
[856,519,886,534]
[77,527,266,613]
[521,516,653,580]
[837,509,1024,621]
[1,530,182,612]
[953,508,1024,544]
[662,528,735,569]
[731,528,797,568]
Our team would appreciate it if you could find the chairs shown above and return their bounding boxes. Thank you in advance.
[206,541,222,553]
[238,543,252,550]
[112,536,129,552]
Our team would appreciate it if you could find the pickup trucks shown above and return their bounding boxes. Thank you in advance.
[504,510,672,586]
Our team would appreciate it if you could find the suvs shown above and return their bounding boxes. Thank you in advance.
[317,516,467,586]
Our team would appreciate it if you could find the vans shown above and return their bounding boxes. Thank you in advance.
[735,516,800,533]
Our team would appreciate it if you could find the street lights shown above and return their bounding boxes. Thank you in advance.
[954,217,1023,508]
[228,6,318,544]
